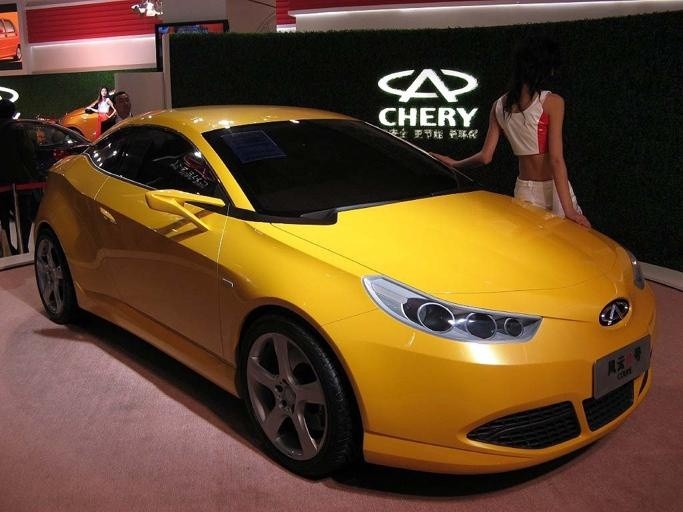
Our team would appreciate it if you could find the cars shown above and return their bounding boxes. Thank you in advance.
[32,105,656,482]
[15,100,103,173]
[0,18,22,62]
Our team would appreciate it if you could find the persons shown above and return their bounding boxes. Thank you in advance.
[101,91,133,134]
[429,36,593,227]
[0,100,40,256]
[87,86,117,139]
